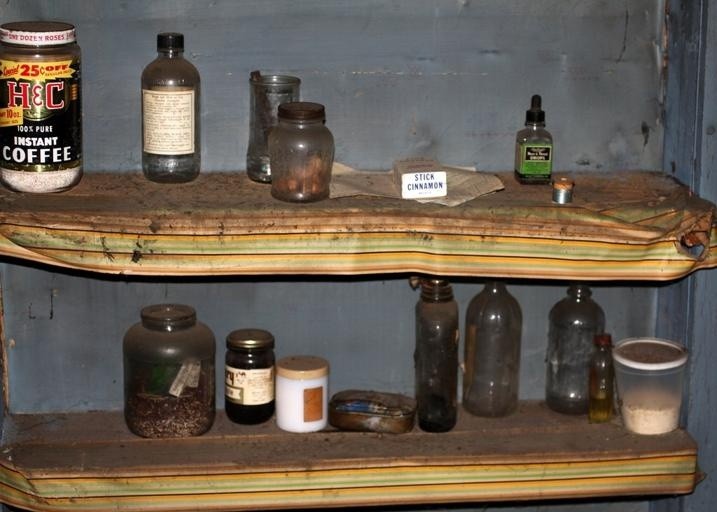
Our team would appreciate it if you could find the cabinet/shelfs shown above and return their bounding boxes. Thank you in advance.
[0,166,715,511]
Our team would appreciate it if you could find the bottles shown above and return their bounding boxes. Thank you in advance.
[541,283,602,417]
[462,284,523,421]
[140,29,203,185]
[1,19,86,189]
[588,332,619,425]
[226,330,279,426]
[271,355,328,435]
[267,101,337,202]
[412,277,458,438]
[117,300,220,437]
[514,93,553,182]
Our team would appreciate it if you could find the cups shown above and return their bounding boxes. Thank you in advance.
[245,72,301,185]
[610,334,690,433]
